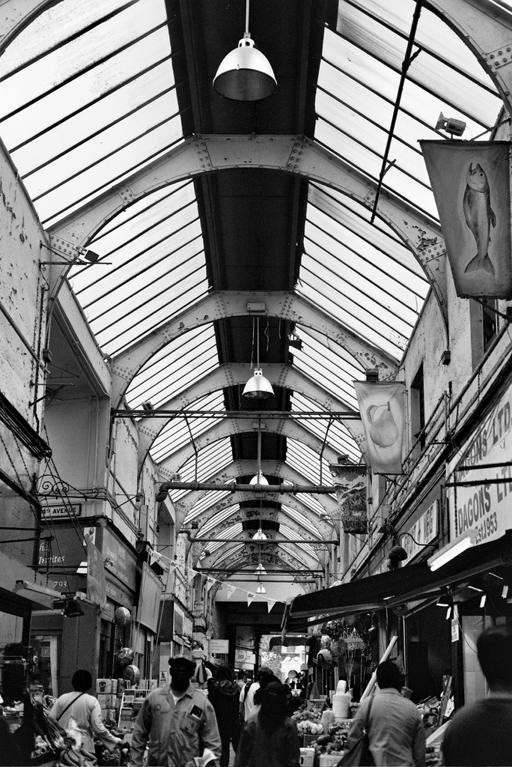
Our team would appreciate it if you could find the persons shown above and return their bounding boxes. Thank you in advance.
[1,620,510,767]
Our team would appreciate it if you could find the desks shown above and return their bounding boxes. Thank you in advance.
[302,734,322,746]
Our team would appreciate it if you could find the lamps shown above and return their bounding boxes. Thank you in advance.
[211,0,277,102]
[242,315,274,400]
[256,575,266,594]
[467,574,483,592]
[251,501,268,540]
[250,416,271,486]
[436,586,450,608]
[489,565,504,581]
[254,543,266,575]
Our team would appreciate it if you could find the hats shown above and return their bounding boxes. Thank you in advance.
[168,657,196,668]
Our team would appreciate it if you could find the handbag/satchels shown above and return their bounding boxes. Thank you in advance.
[336,735,374,767]
